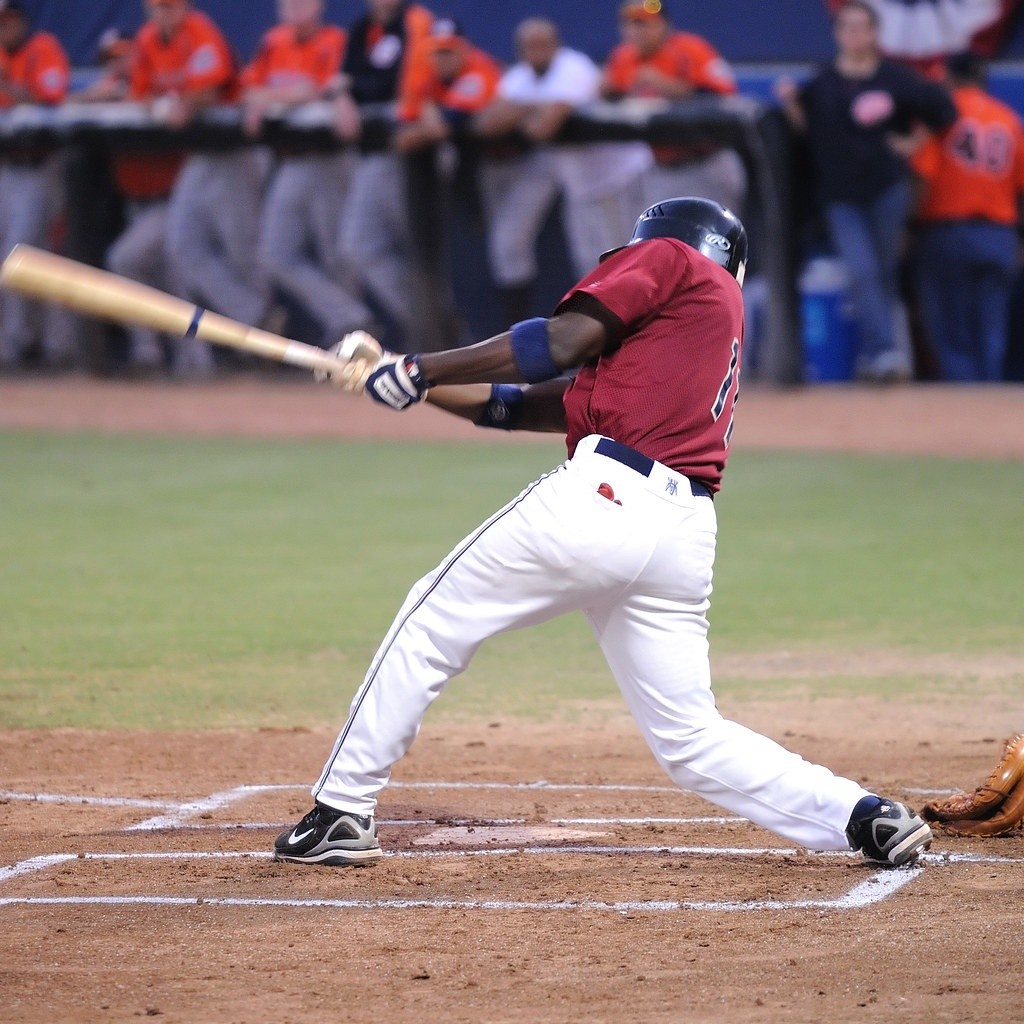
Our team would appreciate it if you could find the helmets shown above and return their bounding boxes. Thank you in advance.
[599,196,748,289]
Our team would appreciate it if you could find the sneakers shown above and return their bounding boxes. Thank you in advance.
[844,797,934,866]
[274,803,383,867]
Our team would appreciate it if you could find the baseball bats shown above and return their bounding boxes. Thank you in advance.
[2,243,430,407]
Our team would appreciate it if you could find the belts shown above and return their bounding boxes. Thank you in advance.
[593,438,715,498]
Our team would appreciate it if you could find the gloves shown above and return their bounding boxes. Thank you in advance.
[314,329,406,395]
[364,349,437,412]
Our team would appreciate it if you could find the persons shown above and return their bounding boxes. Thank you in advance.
[0,0,1024,381]
[274,198,935,867]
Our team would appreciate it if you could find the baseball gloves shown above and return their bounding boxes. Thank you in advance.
[919,727,1023,837]
